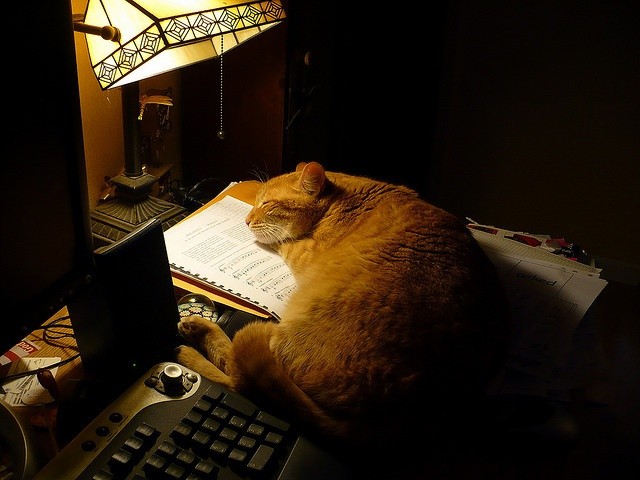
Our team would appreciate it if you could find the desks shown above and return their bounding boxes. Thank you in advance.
[0,180,609,480]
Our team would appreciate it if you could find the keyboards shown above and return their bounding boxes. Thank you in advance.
[30,362,348,479]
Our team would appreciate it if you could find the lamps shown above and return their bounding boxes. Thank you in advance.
[71,0,287,248]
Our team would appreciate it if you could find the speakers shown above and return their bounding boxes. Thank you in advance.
[64,217,181,390]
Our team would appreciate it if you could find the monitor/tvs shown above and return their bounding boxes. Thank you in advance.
[0,0,99,357]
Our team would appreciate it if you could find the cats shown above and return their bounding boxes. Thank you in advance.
[161,162,519,457]
